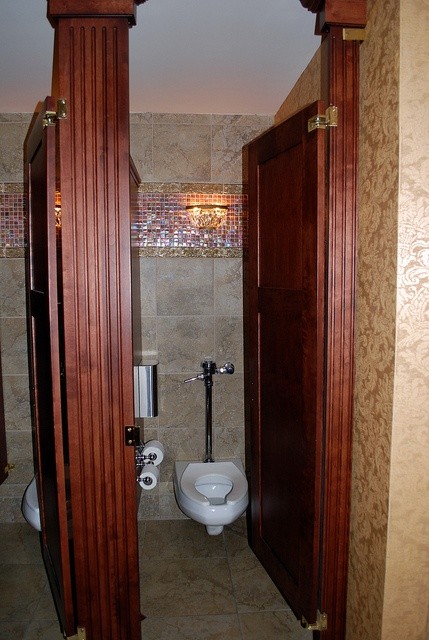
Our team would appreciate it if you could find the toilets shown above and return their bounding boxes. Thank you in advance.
[174,456,249,539]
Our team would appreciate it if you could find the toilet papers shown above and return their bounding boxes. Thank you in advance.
[140,464,160,490]
